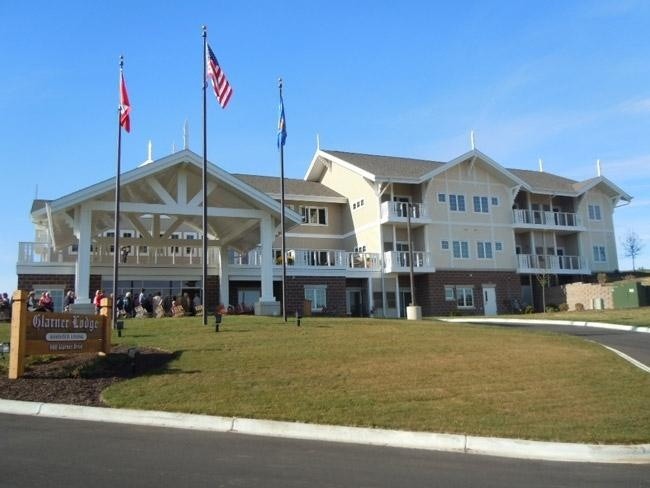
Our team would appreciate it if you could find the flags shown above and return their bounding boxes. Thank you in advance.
[204,42,234,108]
[277,89,288,147]
[119,71,130,131]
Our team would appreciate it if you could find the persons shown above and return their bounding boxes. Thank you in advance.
[64,291,74,306]
[117,289,200,316]
[0,291,54,311]
[94,290,103,314]
